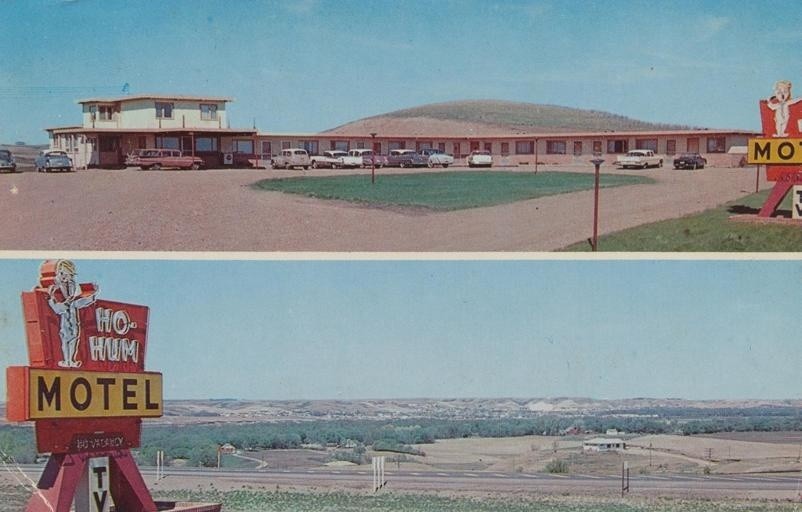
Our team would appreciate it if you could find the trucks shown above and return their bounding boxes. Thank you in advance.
[122,147,204,171]
[560,425,592,436]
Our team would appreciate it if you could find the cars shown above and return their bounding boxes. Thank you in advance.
[0,149,17,173]
[614,149,665,170]
[270,147,455,171]
[35,149,74,173]
[674,152,708,170]
[467,149,493,168]
